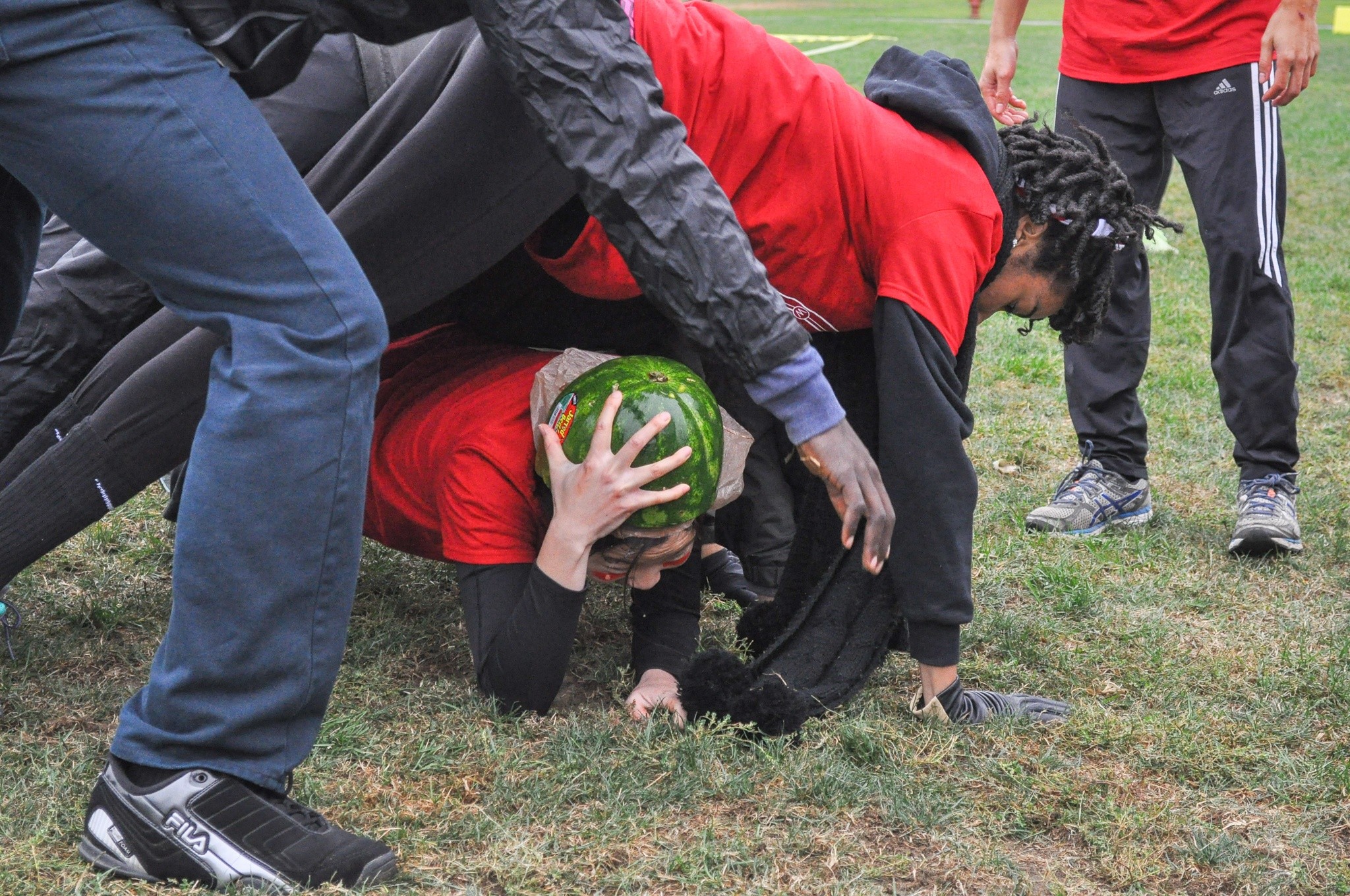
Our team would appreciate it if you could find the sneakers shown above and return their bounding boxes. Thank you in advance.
[1025,439,1154,538]
[1228,472,1303,560]
[77,754,400,893]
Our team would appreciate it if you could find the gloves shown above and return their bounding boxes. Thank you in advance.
[699,546,778,607]
[910,678,1074,724]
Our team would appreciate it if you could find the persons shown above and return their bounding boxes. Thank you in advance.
[0,0,1184,730]
[979,2,1320,557]
[354,314,753,734]
[0,0,898,896]
